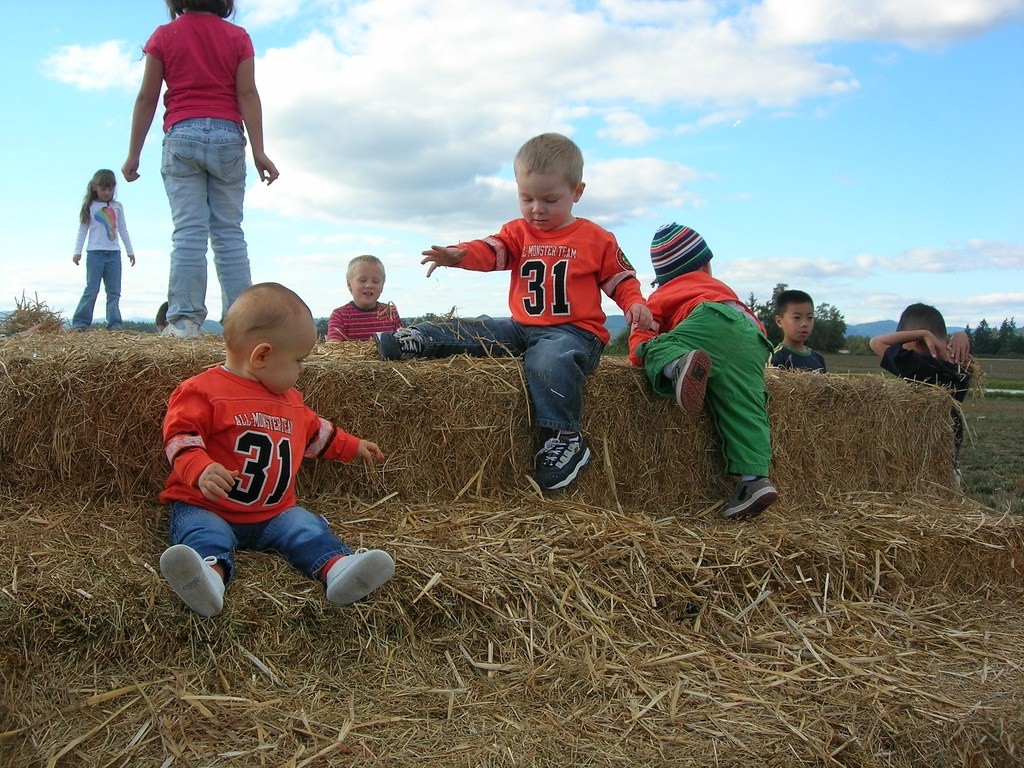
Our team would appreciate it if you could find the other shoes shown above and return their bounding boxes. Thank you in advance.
[160,315,201,341]
[326,546,396,606]
[159,543,226,617]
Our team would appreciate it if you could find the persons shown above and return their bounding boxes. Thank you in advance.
[121,0,280,339]
[374,132,653,490]
[71,170,136,332]
[156,302,168,330]
[327,255,404,341]
[765,289,827,372]
[628,224,778,518]
[159,283,394,617]
[870,304,975,451]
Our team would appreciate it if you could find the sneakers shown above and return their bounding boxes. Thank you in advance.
[670,349,713,419]
[532,429,591,490]
[723,476,779,520]
[374,327,425,362]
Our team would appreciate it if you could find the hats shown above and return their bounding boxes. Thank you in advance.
[651,221,714,286]
